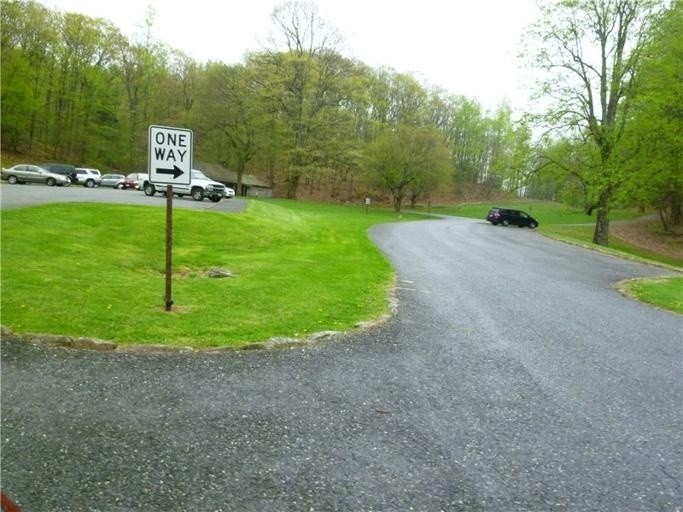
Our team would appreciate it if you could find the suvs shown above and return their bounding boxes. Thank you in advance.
[485,208,538,229]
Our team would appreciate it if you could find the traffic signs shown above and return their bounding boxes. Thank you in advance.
[149,124,194,187]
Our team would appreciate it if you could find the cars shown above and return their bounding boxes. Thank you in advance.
[0,160,236,202]
